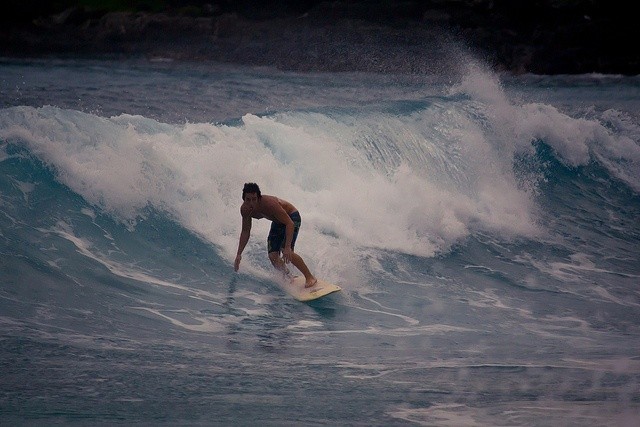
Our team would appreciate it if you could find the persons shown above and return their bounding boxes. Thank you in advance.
[233,184,317,288]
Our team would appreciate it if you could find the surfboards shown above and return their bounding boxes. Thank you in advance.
[279,274,341,302]
